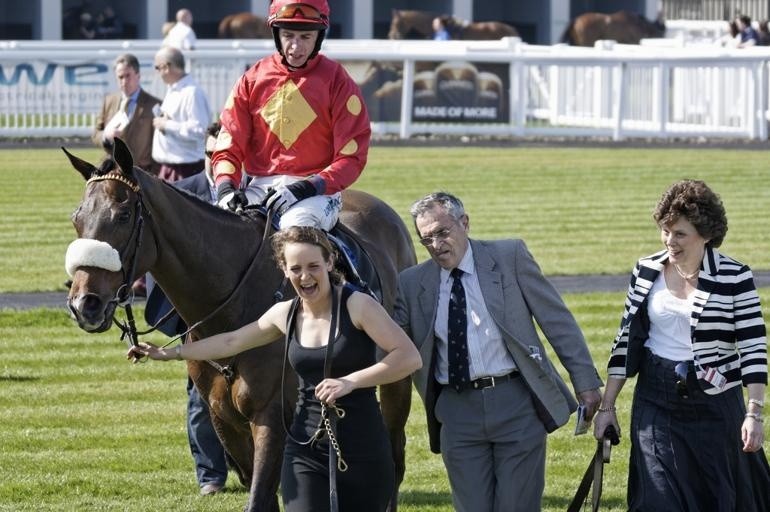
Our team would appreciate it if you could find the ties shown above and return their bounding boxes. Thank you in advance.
[122,98,131,112]
[447,268,471,393]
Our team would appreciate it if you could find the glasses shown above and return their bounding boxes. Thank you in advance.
[674,362,692,399]
[419,219,461,246]
[271,2,325,20]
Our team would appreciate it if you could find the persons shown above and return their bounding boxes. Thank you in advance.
[63,53,162,297]
[95,6,124,38]
[717,14,770,48]
[124,223,424,512]
[74,12,96,39]
[591,179,769,512]
[163,21,175,37]
[141,120,226,495]
[431,14,452,40]
[390,192,605,511]
[146,45,213,183]
[163,8,200,76]
[203,0,373,289]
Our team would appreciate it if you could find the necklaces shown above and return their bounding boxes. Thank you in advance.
[673,263,700,280]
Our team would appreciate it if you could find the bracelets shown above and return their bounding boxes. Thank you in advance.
[595,404,619,414]
[744,411,766,422]
[175,342,184,361]
[747,398,767,409]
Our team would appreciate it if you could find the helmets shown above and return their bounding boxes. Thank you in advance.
[268,1,330,31]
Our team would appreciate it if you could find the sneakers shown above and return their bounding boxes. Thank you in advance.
[201,484,222,495]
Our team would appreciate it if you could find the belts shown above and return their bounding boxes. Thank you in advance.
[471,372,515,389]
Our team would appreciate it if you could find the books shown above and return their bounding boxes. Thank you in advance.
[151,102,166,135]
[103,111,130,133]
[573,405,590,437]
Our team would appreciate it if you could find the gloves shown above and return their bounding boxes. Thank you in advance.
[262,180,313,212]
[216,181,248,210]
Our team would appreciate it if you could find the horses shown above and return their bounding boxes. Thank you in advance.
[59,135,419,512]
[387,8,519,41]
[218,13,274,39]
[560,9,665,47]
